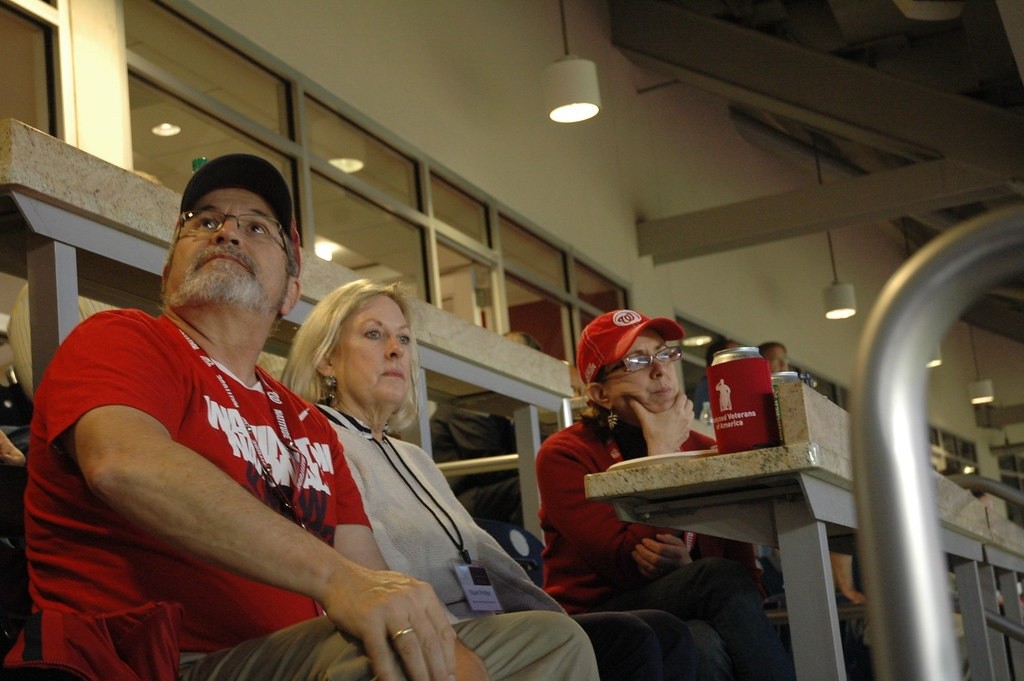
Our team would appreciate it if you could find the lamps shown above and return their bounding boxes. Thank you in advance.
[967,379,994,405]
[926,344,942,369]
[539,58,602,125]
[821,283,857,319]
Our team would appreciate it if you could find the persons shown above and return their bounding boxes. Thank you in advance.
[431,331,543,530]
[0,331,35,468]
[280,280,695,681]
[23,154,600,681]
[705,336,788,375]
[536,308,796,681]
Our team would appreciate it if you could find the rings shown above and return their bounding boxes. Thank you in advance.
[390,625,414,642]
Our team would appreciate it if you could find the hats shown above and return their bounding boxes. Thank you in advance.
[179,152,301,265]
[576,310,686,385]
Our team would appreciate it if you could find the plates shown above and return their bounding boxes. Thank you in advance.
[605,450,717,471]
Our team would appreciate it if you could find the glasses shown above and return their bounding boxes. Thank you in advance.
[767,357,790,364]
[602,346,683,380]
[170,209,294,268]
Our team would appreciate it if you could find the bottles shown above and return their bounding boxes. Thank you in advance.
[699,402,713,429]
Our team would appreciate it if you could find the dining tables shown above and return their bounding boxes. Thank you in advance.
[978,508,1023,681]
[582,376,995,681]
[0,117,575,553]
